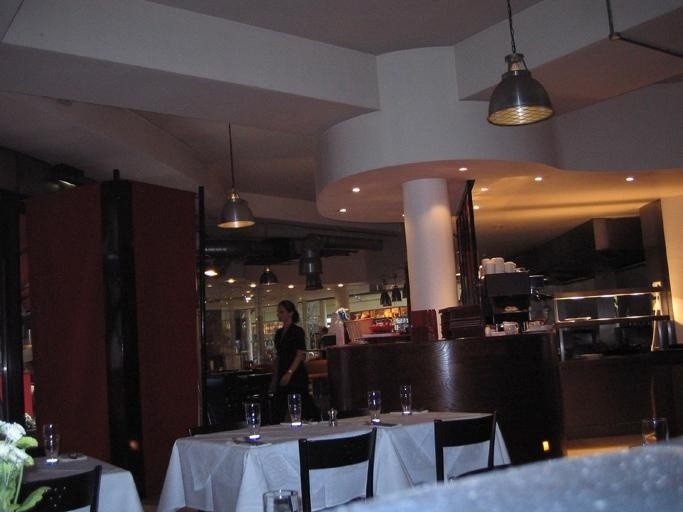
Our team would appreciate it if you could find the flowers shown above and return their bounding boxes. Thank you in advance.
[0,420,51,511]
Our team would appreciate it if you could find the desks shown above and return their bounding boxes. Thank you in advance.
[18,450,136,511]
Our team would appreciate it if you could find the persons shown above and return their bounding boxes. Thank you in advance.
[272,300,308,419]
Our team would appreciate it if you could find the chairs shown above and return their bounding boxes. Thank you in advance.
[298,427,377,511]
[434,410,498,483]
[17,461,102,512]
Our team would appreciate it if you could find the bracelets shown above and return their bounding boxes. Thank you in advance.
[287,369,293,375]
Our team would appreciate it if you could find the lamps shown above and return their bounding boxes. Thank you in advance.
[486,0,556,126]
[218,122,256,228]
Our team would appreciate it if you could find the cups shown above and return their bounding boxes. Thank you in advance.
[43,424,60,461]
[288,393,302,426]
[400,385,413,416]
[481,257,517,275]
[640,416,670,447]
[262,489,302,512]
[368,390,382,424]
[245,402,262,441]
